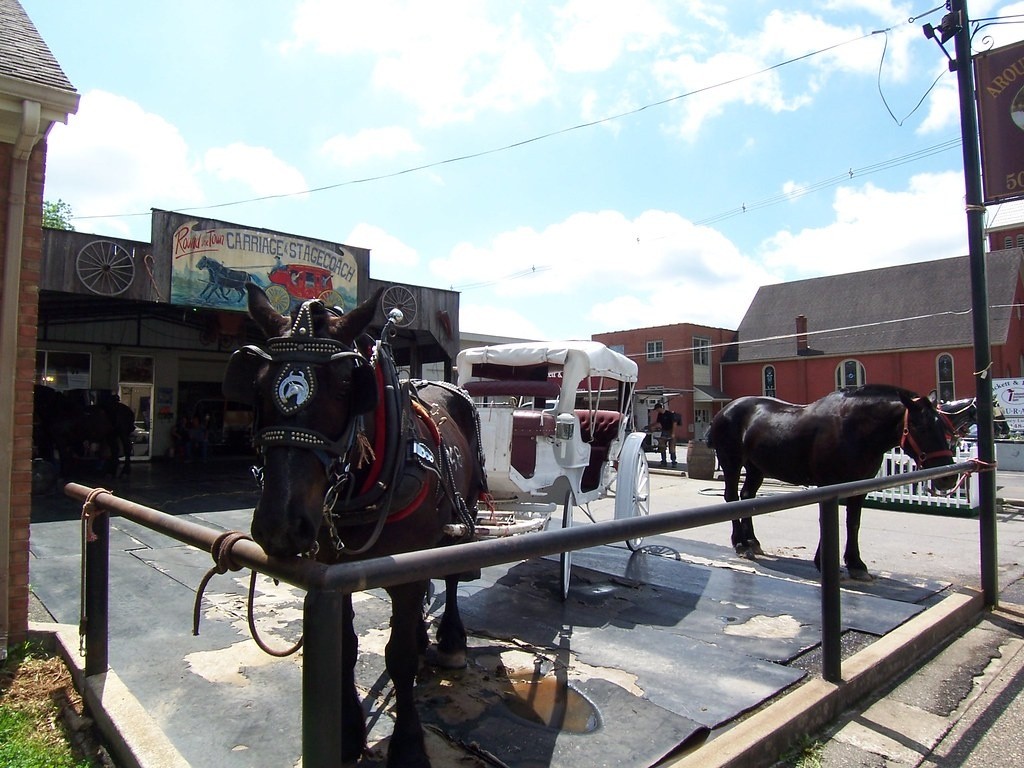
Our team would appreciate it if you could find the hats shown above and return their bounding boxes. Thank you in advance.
[653,403,662,410]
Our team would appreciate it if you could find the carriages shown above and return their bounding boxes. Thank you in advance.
[223,281,650,768]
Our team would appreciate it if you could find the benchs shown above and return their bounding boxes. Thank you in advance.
[574,410,627,456]
[461,380,562,439]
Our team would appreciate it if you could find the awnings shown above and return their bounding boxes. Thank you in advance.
[634,389,698,404]
[693,386,733,402]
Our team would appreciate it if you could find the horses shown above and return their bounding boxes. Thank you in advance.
[887,393,1010,475]
[31,402,142,479]
[709,384,958,582]
[242,279,486,768]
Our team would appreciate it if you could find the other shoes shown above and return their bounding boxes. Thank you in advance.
[672,462,677,468]
[658,460,667,467]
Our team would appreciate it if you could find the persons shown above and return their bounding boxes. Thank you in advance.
[83,393,135,496]
[645,402,678,468]
[172,410,213,465]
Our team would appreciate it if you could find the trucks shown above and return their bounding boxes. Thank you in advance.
[519,386,696,453]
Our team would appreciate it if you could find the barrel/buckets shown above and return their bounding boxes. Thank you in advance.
[686,440,715,479]
